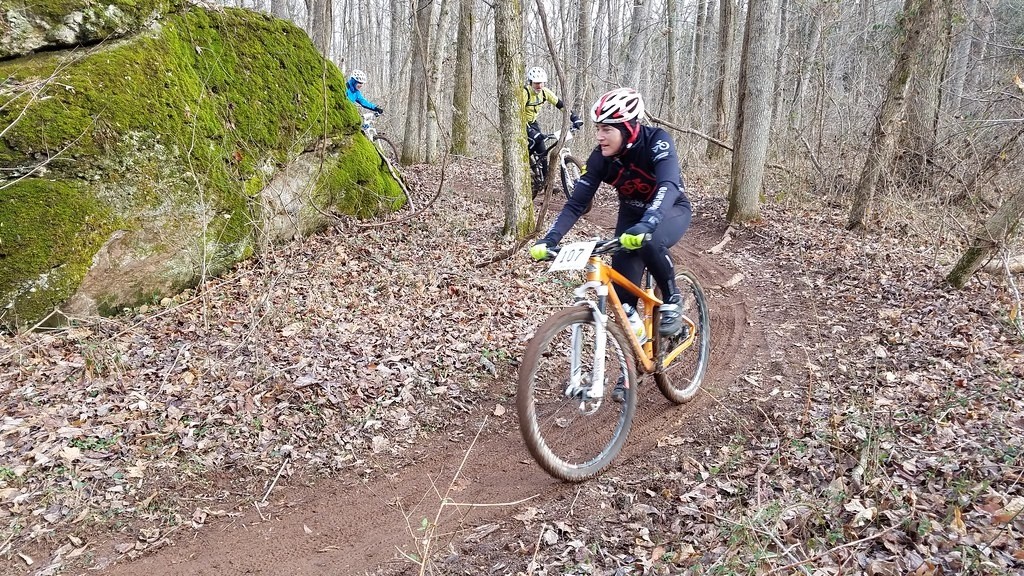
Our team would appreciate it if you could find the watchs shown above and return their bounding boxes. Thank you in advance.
[639,214,660,228]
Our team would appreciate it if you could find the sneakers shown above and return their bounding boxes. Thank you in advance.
[544,185,563,195]
[659,298,684,336]
[611,360,644,403]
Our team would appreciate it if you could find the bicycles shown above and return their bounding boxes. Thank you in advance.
[517,232,711,486]
[528,123,592,215]
[360,109,399,164]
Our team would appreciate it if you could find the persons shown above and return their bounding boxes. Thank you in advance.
[345,69,385,116]
[522,66,585,196]
[525,88,694,404]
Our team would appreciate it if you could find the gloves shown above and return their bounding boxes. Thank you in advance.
[373,104,383,114]
[619,222,656,251]
[572,116,583,130]
[530,238,558,261]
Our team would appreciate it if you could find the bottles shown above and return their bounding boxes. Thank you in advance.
[623,303,648,346]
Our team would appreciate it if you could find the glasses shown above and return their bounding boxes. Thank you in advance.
[357,81,364,84]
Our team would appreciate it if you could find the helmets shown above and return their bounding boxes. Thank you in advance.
[528,66,548,83]
[351,69,367,84]
[591,87,646,124]
